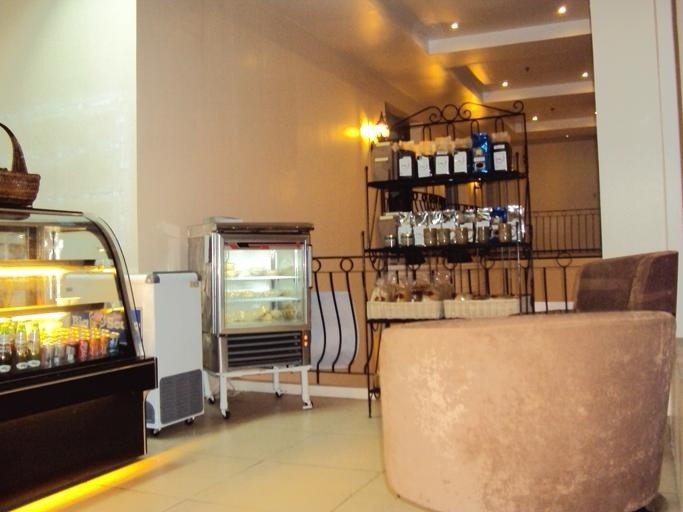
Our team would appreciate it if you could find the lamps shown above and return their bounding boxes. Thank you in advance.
[375,112,388,142]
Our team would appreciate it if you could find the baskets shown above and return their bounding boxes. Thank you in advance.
[0,123,41,206]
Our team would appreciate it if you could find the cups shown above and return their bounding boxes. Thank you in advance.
[423,224,512,244]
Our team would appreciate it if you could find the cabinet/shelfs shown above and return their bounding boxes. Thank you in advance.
[361,170,536,417]
[124,270,205,434]
[0,207,158,512]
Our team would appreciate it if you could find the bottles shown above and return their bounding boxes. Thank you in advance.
[0,319,41,376]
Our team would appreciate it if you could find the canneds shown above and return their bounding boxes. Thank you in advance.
[39,325,121,369]
[401,232,414,246]
[384,235,396,248]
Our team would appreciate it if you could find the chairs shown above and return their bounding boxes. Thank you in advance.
[379,250,678,512]
